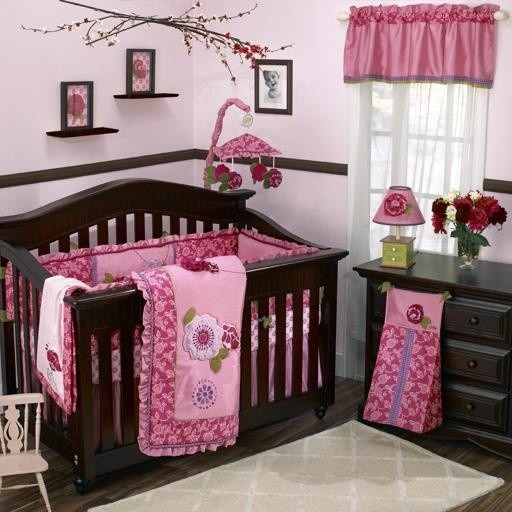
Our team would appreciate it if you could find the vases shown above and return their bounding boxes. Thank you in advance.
[459,242,477,270]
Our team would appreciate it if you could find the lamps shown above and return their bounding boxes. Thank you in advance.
[373,187,424,269]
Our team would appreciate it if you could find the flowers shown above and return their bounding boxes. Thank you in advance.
[20,1,293,87]
[432,190,508,257]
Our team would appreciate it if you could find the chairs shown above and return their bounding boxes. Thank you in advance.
[0,393,51,512]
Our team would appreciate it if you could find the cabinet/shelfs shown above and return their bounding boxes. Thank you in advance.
[353,251,511,461]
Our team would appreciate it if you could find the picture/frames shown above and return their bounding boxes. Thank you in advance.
[61,80,93,131]
[126,49,155,94]
[255,59,293,115]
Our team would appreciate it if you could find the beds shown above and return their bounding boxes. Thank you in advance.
[0,178,350,495]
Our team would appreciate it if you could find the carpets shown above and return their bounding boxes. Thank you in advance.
[86,421,504,511]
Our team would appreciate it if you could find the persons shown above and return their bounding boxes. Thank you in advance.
[262,70,283,104]
